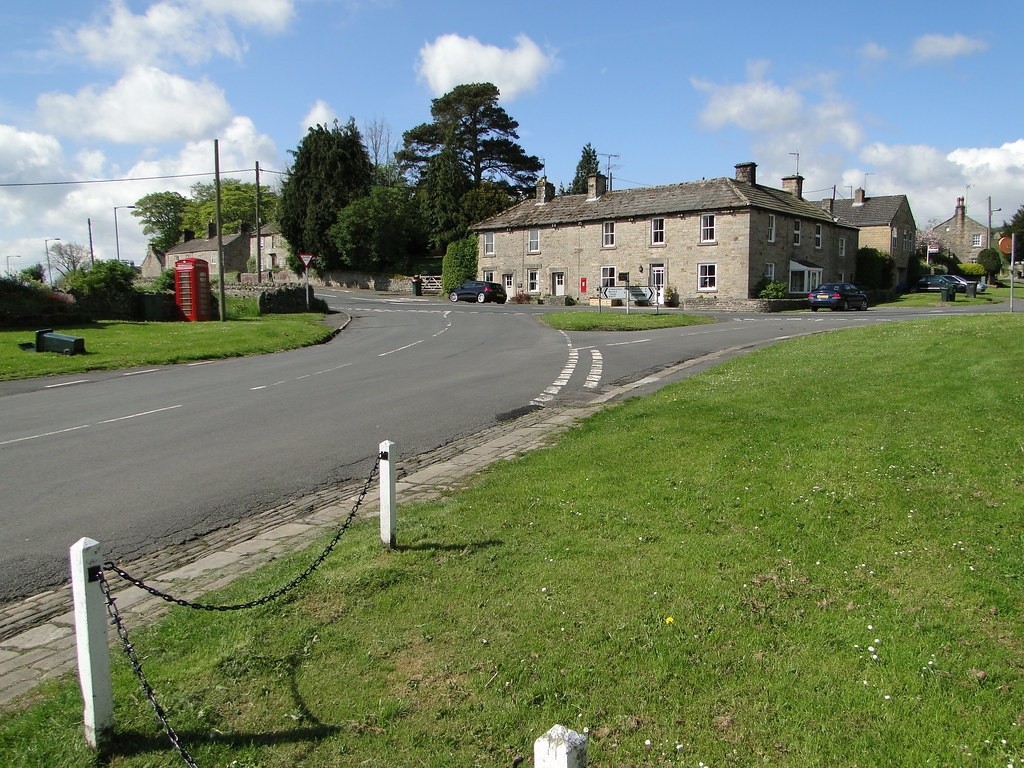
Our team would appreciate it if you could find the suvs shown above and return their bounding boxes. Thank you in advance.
[449,281,507,304]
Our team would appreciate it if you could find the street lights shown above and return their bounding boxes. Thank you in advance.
[45,238,61,287]
[7,255,21,280]
[988,205,1001,246]
[114,206,135,261]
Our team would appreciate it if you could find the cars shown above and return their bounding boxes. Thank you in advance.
[808,282,868,311]
[909,274,987,293]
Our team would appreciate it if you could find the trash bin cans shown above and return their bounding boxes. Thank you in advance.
[966,282,977,298]
[412,281,422,296]
[940,284,956,302]
[17,328,85,356]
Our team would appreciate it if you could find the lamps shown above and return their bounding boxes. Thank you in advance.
[639,265,643,272]
